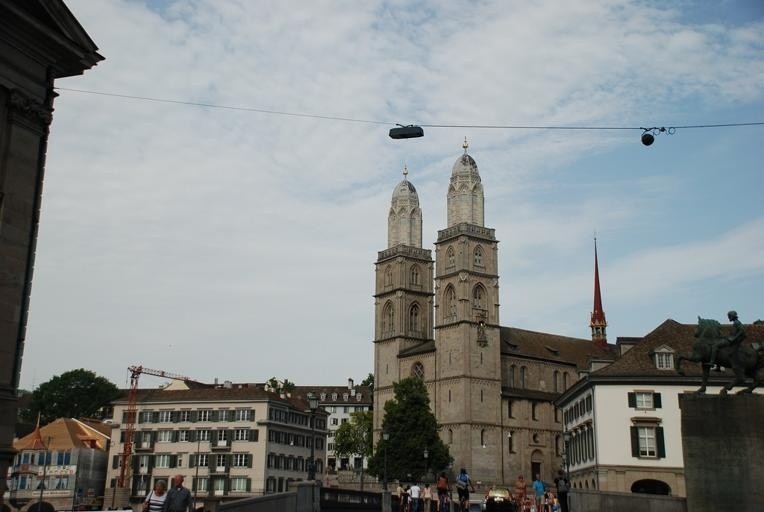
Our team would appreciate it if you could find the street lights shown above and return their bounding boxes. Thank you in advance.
[307,395,319,480]
[563,427,573,487]
[423,446,429,486]
[379,430,390,491]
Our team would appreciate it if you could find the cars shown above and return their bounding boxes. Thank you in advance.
[480,495,488,512]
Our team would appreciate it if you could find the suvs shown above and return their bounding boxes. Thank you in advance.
[484,486,515,512]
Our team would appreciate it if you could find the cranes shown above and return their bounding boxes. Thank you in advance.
[116,364,195,490]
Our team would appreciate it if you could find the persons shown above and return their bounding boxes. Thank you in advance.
[144,479,167,512]
[704,310,747,368]
[395,467,475,511]
[514,473,571,512]
[161,473,195,512]
